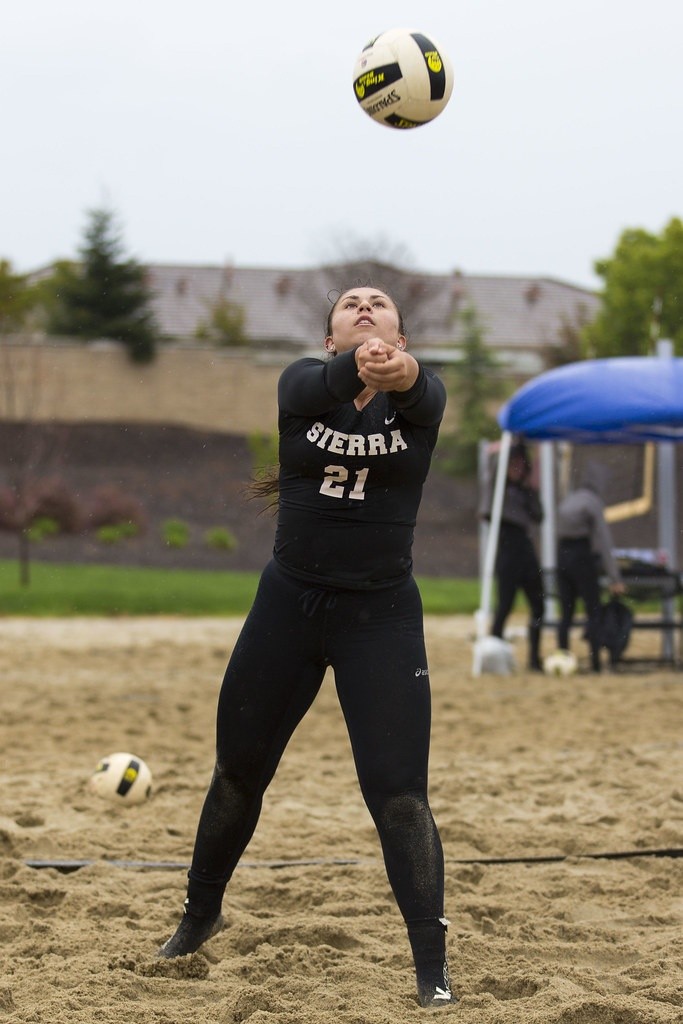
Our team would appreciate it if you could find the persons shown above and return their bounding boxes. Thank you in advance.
[478,433,546,676]
[160,286,455,1006]
[556,470,626,673]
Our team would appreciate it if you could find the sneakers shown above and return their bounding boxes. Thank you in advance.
[416,960,458,1010]
[154,909,224,959]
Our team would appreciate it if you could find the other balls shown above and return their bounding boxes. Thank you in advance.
[543,651,577,677]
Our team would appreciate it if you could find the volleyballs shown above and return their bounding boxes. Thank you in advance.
[352,28,454,130]
[92,749,152,806]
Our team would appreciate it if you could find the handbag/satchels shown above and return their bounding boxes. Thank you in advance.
[583,594,634,654]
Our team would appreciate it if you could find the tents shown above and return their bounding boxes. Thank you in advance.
[472,355,683,678]
